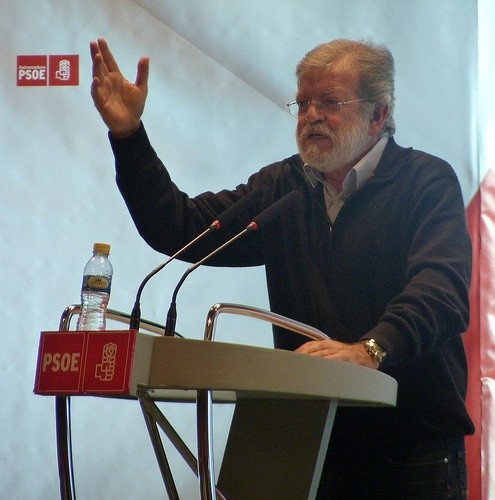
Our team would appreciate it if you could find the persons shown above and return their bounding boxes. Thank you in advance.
[89,38,475,500]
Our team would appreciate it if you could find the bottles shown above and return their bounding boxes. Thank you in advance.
[75,243,114,331]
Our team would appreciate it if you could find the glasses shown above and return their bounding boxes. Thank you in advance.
[286,99,366,120]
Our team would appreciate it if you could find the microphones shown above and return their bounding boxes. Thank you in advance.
[164,190,304,338]
[129,187,266,329]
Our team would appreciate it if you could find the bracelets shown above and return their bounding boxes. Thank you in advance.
[360,338,387,364]
